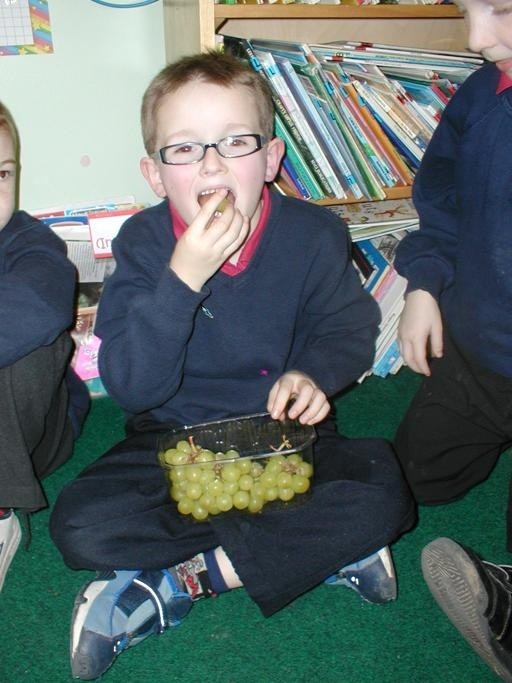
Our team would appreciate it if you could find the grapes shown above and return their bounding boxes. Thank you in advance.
[216,199,229,212]
[157,440,313,521]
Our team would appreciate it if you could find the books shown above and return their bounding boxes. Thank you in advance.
[31,201,144,283]
[324,202,420,384]
[235,40,486,200]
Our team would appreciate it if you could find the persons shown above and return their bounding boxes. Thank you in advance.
[49,45,420,683]
[393,0,512,507]
[0,103,91,591]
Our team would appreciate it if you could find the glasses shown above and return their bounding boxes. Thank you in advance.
[148,133,270,164]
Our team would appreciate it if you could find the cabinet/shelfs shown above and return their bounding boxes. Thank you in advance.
[164,0,488,370]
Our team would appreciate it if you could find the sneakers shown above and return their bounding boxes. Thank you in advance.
[69,560,193,678]
[325,544,396,604]
